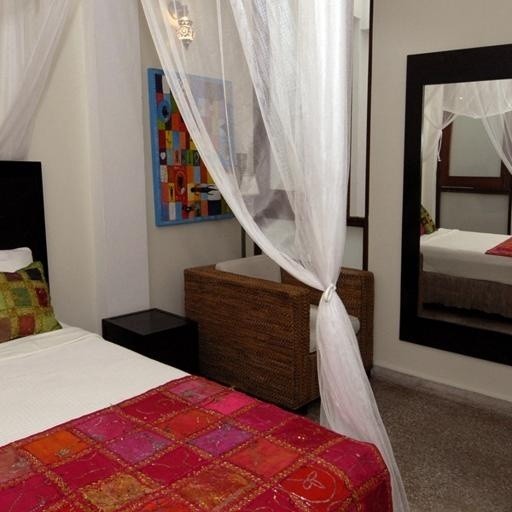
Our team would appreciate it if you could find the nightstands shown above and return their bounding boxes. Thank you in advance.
[103,309,202,377]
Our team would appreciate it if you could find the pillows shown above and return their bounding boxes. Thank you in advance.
[421,206,436,235]
[1,261,61,345]
[0,246,36,274]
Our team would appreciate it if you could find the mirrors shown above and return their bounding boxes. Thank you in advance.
[400,42,512,367]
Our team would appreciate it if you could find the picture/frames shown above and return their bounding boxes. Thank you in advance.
[162,0,193,51]
[147,66,240,227]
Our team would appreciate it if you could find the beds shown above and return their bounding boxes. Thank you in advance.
[1,160,392,511]
[420,228,512,320]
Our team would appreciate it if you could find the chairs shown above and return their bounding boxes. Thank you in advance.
[184,254,374,410]
